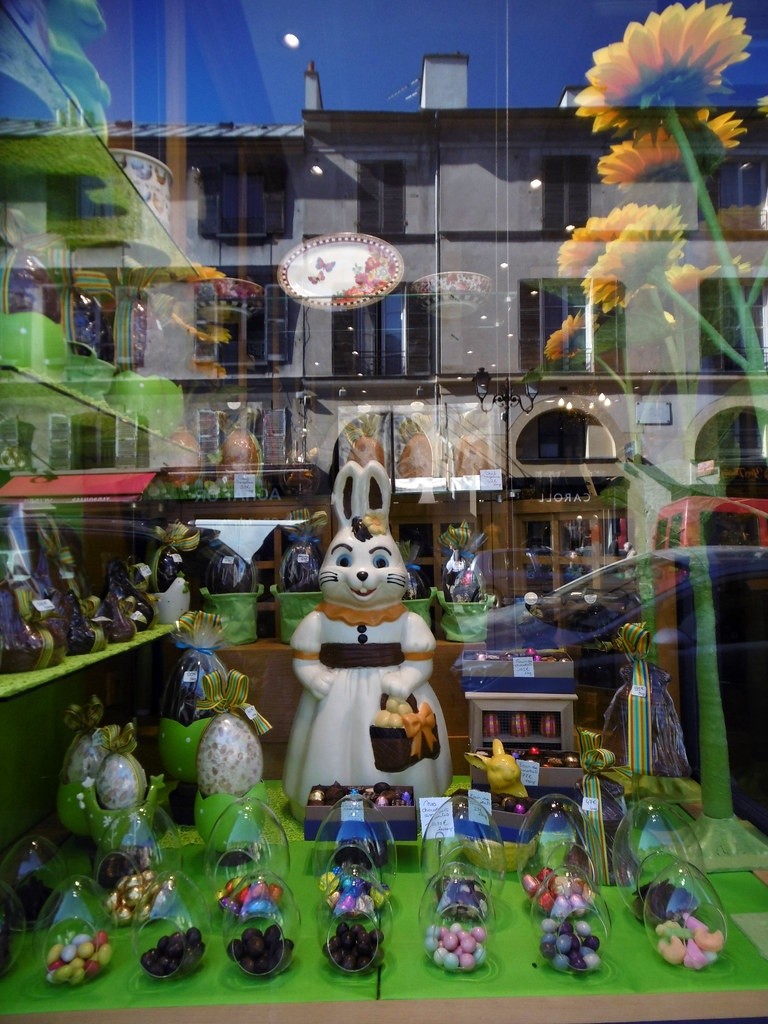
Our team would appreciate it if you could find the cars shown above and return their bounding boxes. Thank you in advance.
[468,544,768,771]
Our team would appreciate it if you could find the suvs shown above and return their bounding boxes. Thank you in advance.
[2,516,274,602]
[450,547,582,606]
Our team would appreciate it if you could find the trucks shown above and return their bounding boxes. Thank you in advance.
[186,519,303,585]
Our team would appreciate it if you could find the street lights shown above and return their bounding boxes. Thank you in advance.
[471,366,543,603]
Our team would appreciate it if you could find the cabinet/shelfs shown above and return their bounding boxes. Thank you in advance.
[463,692,580,794]
[1,3,208,710]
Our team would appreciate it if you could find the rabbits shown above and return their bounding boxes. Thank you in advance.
[291,460,453,823]
[463,738,527,798]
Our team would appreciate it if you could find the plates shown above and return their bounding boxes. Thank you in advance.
[277,232,404,311]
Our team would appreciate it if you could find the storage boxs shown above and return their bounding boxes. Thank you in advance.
[302,785,419,843]
[470,748,582,794]
[456,806,545,843]
[463,649,574,692]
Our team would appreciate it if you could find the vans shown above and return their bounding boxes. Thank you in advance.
[656,496,768,548]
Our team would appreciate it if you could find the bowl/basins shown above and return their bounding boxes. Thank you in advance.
[412,272,492,318]
[193,278,267,324]
[76,149,173,269]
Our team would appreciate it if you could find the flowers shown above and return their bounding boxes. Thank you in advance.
[541,1,767,485]
